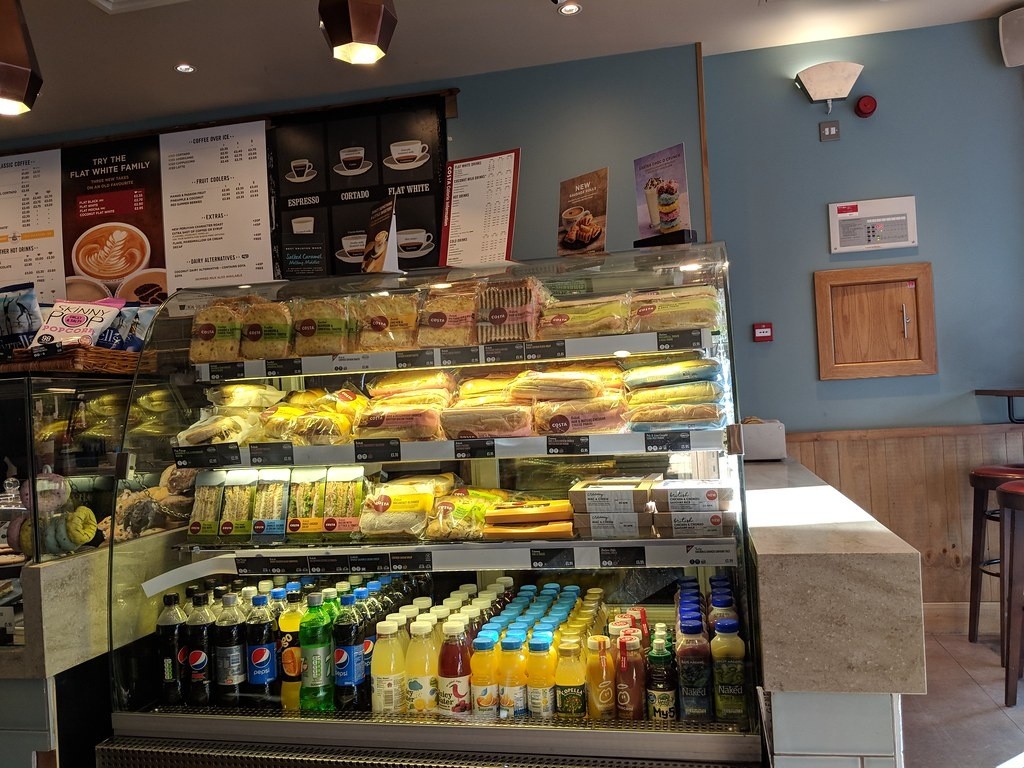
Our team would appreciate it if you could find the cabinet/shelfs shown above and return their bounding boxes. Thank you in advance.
[107,240,763,765]
[0,371,201,768]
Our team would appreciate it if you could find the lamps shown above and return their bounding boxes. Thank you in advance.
[317,0,399,65]
[0,0,44,117]
[795,60,864,116]
[550,0,583,17]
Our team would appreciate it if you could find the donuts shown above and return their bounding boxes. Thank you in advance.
[656,179,680,233]
[6,472,97,555]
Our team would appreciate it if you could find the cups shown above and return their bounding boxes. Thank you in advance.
[646,178,670,234]
[135,267,140,271]
[292,156,312,173]
[396,226,430,245]
[338,147,369,169]
[345,232,371,254]
[63,221,166,304]
[562,203,596,229]
[388,139,430,161]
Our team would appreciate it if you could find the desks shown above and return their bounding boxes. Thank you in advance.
[975,387,1024,424]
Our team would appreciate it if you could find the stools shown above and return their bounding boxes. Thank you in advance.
[995,480,1024,708]
[968,466,1024,644]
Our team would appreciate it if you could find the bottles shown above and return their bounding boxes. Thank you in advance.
[150,574,302,710]
[506,585,576,616]
[677,573,748,723]
[435,577,508,616]
[435,616,595,718]
[587,636,613,722]
[613,607,678,721]
[579,587,610,614]
[305,573,436,713]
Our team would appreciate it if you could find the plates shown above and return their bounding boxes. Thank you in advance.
[383,155,432,169]
[336,251,373,266]
[284,171,318,183]
[328,166,378,176]
[390,243,442,261]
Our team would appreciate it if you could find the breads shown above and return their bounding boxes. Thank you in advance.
[96,463,197,550]
[36,381,191,448]
[357,474,510,540]
[563,214,601,243]
[184,276,722,444]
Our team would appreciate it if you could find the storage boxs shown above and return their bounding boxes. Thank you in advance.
[482,520,578,541]
[485,500,574,524]
[568,478,651,512]
[573,506,655,540]
[728,418,789,464]
[654,507,737,539]
[651,479,735,512]
[591,472,664,481]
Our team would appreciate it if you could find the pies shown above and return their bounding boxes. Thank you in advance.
[0,543,26,565]
